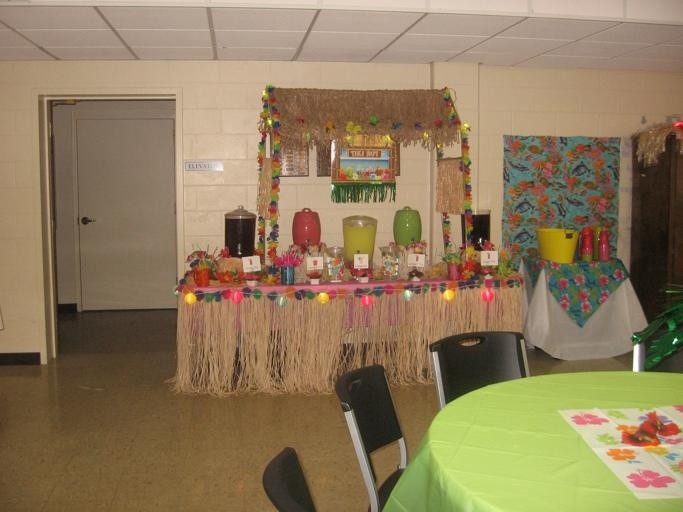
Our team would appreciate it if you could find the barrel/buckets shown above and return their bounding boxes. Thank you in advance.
[536,229,579,264]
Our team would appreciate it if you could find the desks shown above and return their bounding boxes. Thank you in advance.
[173,272,526,398]
[519,254,648,362]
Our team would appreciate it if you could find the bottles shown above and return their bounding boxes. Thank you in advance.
[581,226,593,241]
[598,230,610,262]
[580,235,594,264]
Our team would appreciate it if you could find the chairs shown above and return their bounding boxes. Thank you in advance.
[334,364,409,512]
[262,447,316,512]
[430,331,528,409]
